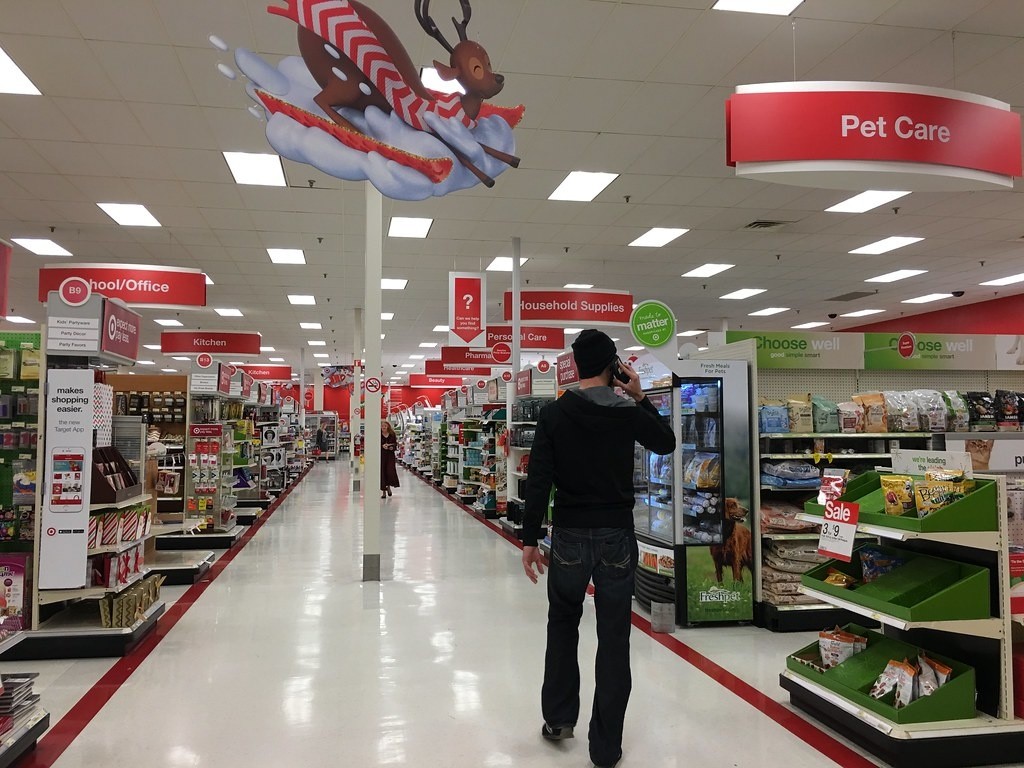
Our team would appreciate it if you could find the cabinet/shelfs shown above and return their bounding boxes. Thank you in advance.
[393,417,550,539]
[0,325,167,662]
[0,631,50,767]
[143,375,351,586]
[689,338,1024,768]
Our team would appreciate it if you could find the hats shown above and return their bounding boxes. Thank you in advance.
[571,329,617,379]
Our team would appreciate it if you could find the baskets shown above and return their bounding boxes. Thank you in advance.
[312,445,321,455]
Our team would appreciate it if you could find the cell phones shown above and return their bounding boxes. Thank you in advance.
[50,446,87,513]
[609,354,631,384]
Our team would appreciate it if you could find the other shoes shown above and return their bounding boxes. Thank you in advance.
[387,487,392,496]
[316,459,319,463]
[381,495,386,498]
[594,748,622,768]
[326,459,329,463]
[542,722,574,739]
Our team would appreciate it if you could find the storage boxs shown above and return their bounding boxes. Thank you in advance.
[89,445,185,628]
[0,552,33,631]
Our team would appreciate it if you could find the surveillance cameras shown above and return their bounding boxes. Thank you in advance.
[393,365,397,367]
[952,290,964,297]
[826,313,839,318]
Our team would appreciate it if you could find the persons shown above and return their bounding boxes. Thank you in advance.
[316,420,330,463]
[523,328,677,768]
[380,421,401,498]
